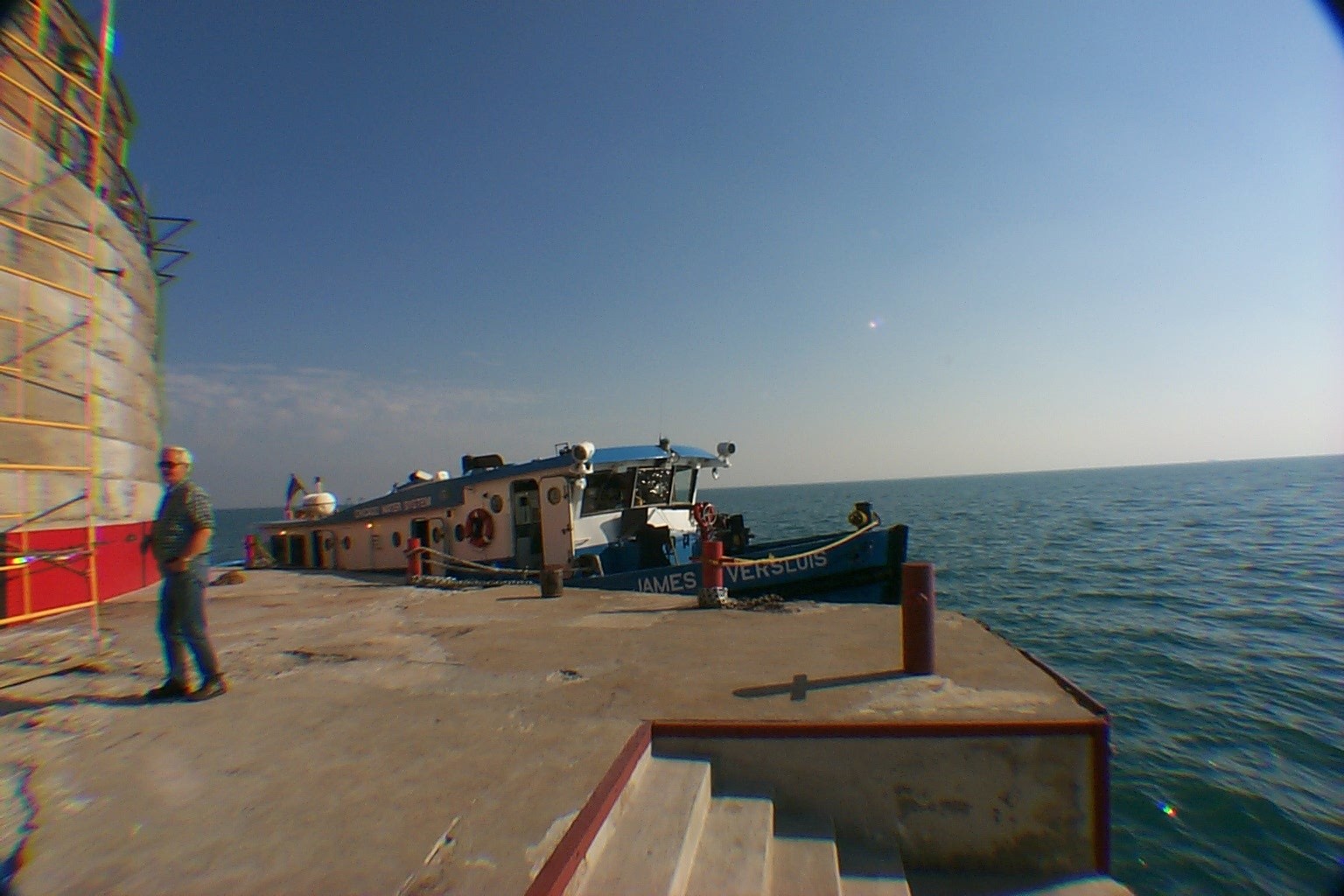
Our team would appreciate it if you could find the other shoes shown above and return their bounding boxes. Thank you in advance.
[189,673,229,702]
[146,679,193,701]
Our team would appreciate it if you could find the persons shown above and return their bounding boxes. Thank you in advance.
[285,508,294,520]
[140,447,228,702]
[315,476,321,493]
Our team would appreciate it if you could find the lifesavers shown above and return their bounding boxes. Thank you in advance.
[696,502,716,530]
[463,510,498,549]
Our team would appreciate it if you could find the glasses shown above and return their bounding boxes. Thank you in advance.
[159,461,187,469]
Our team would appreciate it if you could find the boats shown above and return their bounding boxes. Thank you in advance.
[256,434,910,603]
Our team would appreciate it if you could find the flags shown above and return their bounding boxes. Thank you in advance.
[284,475,301,510]
